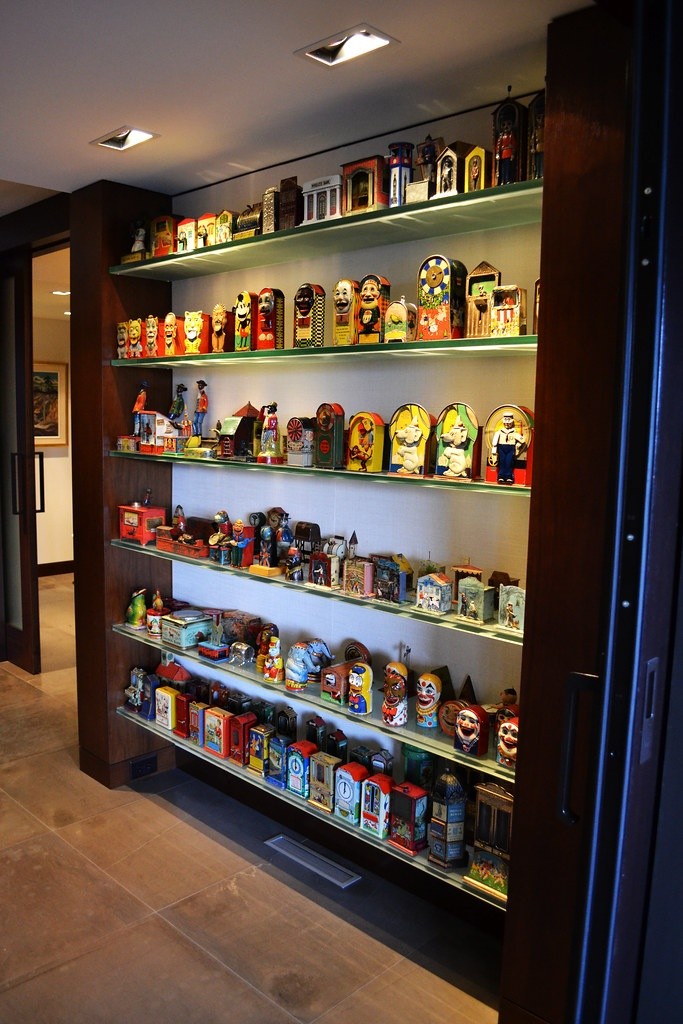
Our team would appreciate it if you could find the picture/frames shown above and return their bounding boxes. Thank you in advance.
[33,361,69,447]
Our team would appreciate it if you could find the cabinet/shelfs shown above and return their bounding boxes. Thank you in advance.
[105,176,569,915]
